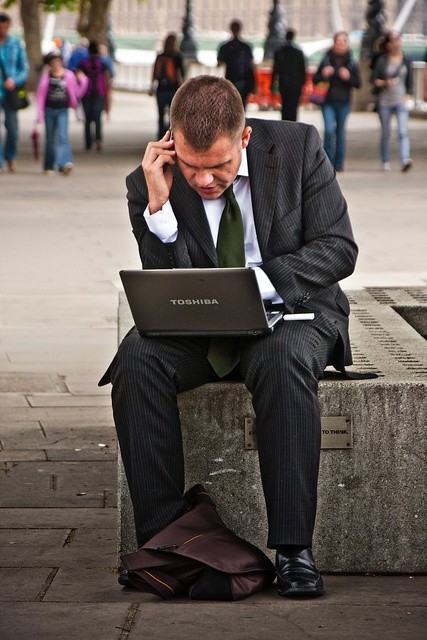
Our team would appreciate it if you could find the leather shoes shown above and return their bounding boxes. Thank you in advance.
[117,570,154,593]
[274,547,324,599]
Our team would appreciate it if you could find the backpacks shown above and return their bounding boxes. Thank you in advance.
[154,55,177,85]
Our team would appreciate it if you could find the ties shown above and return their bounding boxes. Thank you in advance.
[206,183,246,378]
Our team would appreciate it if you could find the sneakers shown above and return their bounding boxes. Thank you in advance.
[45,170,55,174]
[383,162,391,172]
[402,158,413,172]
[63,162,73,175]
[7,160,17,171]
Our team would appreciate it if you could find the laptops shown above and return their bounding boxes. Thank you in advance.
[118,268,284,340]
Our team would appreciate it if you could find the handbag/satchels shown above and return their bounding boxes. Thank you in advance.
[119,483,276,601]
[308,55,330,106]
[0,58,29,111]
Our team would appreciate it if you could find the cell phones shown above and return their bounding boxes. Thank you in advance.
[162,128,176,174]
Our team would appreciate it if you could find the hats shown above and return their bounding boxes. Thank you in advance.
[43,51,63,65]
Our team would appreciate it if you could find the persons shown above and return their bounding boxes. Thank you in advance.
[311,32,363,169]
[77,42,109,151]
[0,12,30,172]
[269,29,308,121]
[216,19,256,116]
[97,74,359,591]
[36,50,88,175]
[371,33,415,173]
[147,34,185,142]
